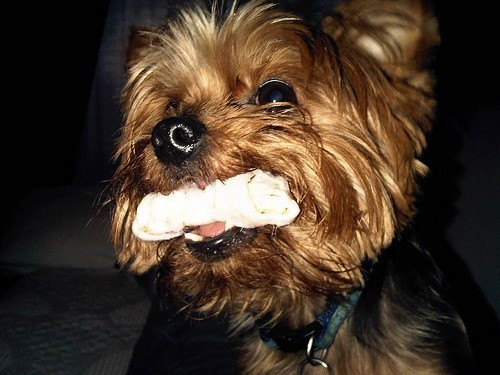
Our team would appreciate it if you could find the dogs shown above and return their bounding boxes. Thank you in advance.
[83,0,500,375]
